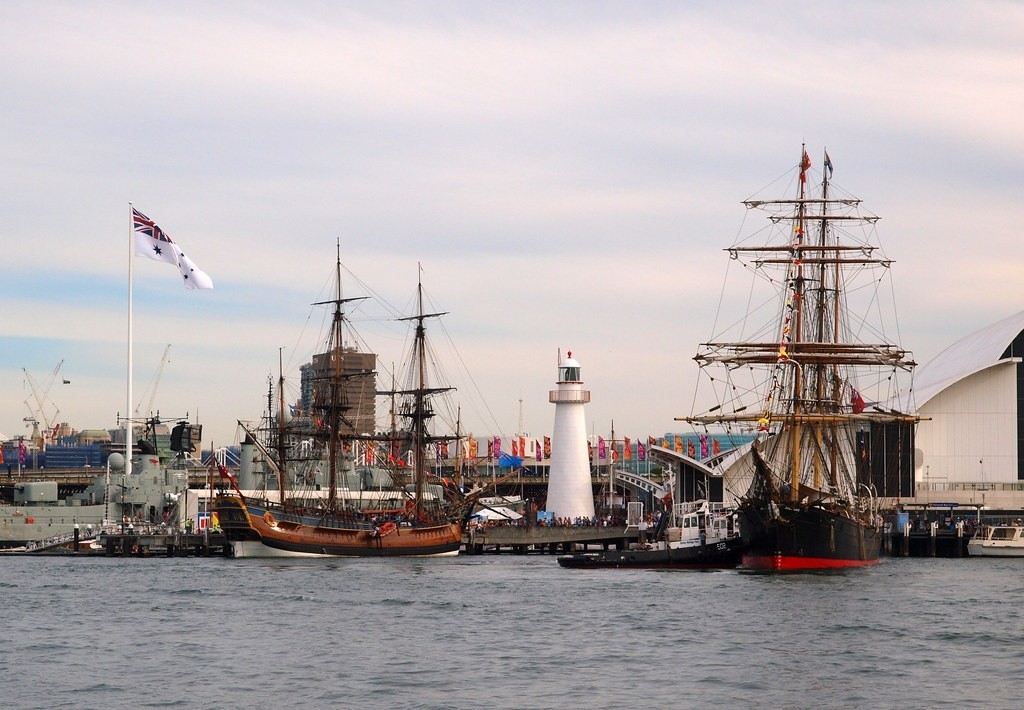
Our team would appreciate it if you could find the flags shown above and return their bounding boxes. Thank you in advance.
[288,406,448,486]
[850,387,866,462]
[756,227,805,431]
[438,433,720,463]
[797,150,811,184]
[824,151,834,178]
[217,463,239,492]
[132,209,213,291]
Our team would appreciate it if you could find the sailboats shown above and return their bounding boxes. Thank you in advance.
[215,236,527,557]
[670,136,932,574]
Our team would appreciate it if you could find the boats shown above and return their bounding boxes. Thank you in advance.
[556,534,742,572]
[967,524,1024,557]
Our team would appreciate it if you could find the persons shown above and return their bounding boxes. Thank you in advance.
[185,517,194,534]
[477,509,662,525]
